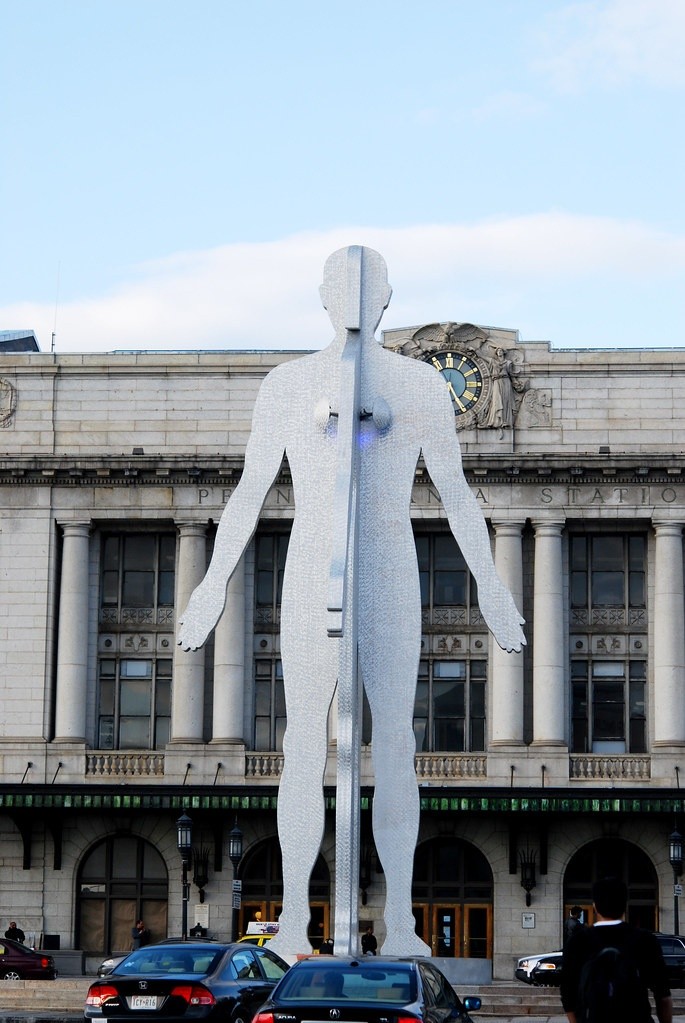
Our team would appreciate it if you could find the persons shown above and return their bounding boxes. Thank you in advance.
[131,920,150,952]
[559,878,674,1023]
[360,928,377,955]
[5,921,25,944]
[393,345,404,353]
[483,346,519,427]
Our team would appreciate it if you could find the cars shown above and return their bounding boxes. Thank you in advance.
[0,938,59,980]
[250,953,483,1023]
[82,943,295,1023]
[514,930,685,987]
[96,937,221,978]
[194,935,277,971]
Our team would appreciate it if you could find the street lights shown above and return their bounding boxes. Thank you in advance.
[229,816,243,948]
[669,830,682,938]
[175,813,194,941]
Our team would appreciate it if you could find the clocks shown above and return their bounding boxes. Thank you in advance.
[422,346,491,425]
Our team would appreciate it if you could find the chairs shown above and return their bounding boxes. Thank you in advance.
[323,972,347,998]
[174,954,195,972]
[193,959,211,972]
[391,983,410,999]
[139,962,158,973]
[230,959,249,978]
